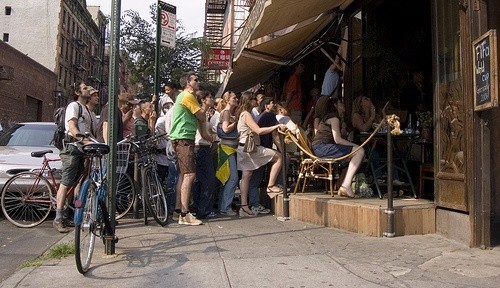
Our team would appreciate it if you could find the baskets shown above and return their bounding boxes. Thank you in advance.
[116,143,131,174]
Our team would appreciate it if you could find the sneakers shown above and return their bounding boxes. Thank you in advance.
[178,212,203,226]
[52,218,68,233]
[65,220,74,227]
[173,211,180,221]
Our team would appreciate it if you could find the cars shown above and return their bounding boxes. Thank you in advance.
[0,121,74,193]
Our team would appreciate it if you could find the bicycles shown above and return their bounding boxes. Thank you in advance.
[0,132,136,228]
[74,132,169,277]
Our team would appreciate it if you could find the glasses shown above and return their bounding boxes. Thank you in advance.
[194,79,199,82]
[228,91,231,99]
[254,97,257,101]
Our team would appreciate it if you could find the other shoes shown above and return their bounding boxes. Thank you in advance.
[220,206,271,218]
[337,185,360,198]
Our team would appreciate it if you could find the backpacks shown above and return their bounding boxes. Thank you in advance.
[49,102,82,149]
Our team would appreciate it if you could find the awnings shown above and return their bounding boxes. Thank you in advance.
[215,0,353,99]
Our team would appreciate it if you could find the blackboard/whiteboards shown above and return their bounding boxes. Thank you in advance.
[471,29,498,112]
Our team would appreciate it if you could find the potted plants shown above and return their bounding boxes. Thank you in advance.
[416,110,434,141]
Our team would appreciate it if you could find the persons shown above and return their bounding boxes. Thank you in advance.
[52,40,433,233]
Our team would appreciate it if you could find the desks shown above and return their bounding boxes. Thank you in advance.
[413,138,435,197]
[355,131,420,199]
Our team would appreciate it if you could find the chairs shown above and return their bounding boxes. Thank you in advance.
[286,124,339,195]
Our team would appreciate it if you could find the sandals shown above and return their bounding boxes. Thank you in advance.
[266,185,290,199]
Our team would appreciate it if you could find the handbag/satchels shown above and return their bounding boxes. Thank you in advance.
[216,121,239,139]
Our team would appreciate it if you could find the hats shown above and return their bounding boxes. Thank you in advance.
[87,85,99,95]
